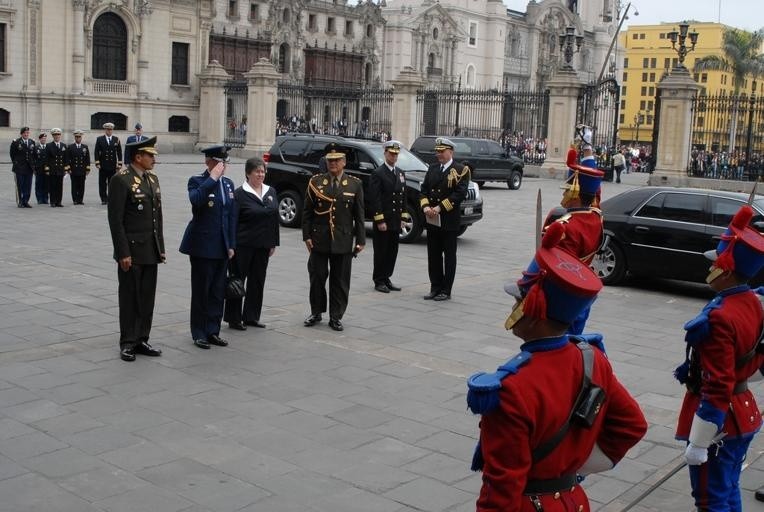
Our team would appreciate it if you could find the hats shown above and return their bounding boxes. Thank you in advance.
[502,245,604,332]
[38,134,48,140]
[432,138,457,151]
[383,140,402,154]
[559,164,605,196]
[702,223,764,281]
[103,121,115,130]
[124,136,160,155]
[324,142,347,159]
[201,145,233,162]
[51,128,62,136]
[134,122,143,130]
[72,130,86,138]
[19,127,30,134]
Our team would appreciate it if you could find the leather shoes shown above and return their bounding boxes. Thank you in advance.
[209,334,228,347]
[134,341,161,357]
[375,283,390,293]
[194,337,211,349]
[386,283,401,291]
[14,200,108,209]
[228,321,247,330]
[119,343,136,362]
[243,321,266,329]
[424,292,439,300]
[304,312,322,326]
[328,318,344,331]
[434,293,448,301]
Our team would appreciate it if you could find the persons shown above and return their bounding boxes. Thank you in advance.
[541,190,605,336]
[301,142,366,330]
[276,113,369,136]
[369,140,406,293]
[229,119,237,137]
[10,127,35,208]
[67,130,91,205]
[418,137,471,301]
[691,146,764,182]
[224,157,280,330]
[675,262,764,512]
[239,118,247,137]
[107,136,168,361]
[44,128,68,207]
[467,299,647,512]
[179,144,237,350]
[503,128,548,166]
[124,123,149,168]
[35,133,49,204]
[94,122,122,205]
[579,141,653,183]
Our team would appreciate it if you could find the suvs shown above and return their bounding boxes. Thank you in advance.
[410,134,524,190]
[259,129,483,243]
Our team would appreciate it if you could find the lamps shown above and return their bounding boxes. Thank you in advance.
[550,20,586,66]
[664,17,700,66]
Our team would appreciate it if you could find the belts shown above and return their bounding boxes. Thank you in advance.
[732,380,748,395]
[523,474,582,494]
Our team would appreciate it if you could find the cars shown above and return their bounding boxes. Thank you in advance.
[540,186,764,302]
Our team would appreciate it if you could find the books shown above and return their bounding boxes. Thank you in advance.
[426,213,442,227]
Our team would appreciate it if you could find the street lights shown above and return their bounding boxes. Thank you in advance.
[633,112,644,146]
[585,2,640,124]
